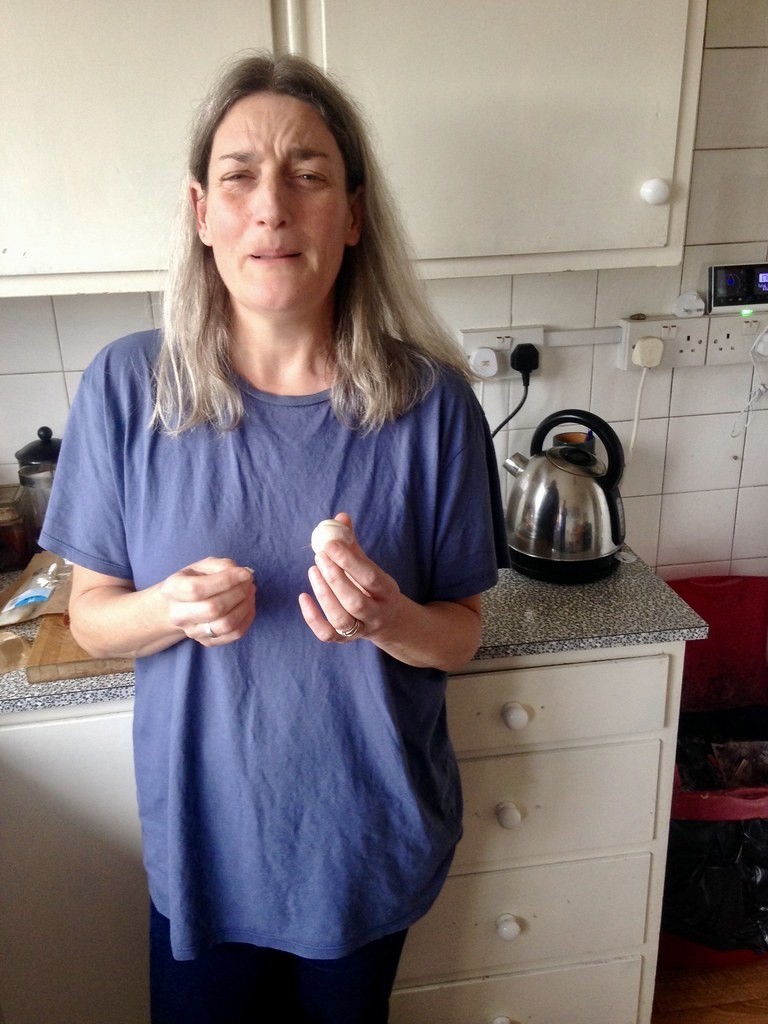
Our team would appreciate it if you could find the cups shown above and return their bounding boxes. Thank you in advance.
[552,430,596,455]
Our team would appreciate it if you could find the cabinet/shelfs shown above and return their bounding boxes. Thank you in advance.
[0,542,708,1024]
[0,1,709,298]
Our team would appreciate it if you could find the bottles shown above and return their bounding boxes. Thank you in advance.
[14,426,64,549]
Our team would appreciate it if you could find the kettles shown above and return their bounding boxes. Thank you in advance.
[498,407,638,584]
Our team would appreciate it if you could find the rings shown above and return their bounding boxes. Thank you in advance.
[205,625,216,640]
[341,622,365,638]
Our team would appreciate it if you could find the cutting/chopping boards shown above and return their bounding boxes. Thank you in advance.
[23,591,133,684]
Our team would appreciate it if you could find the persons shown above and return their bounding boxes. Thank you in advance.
[39,50,509,1024]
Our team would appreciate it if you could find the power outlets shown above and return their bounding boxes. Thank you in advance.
[616,316,709,371]
[706,313,768,366]
[458,325,544,383]
